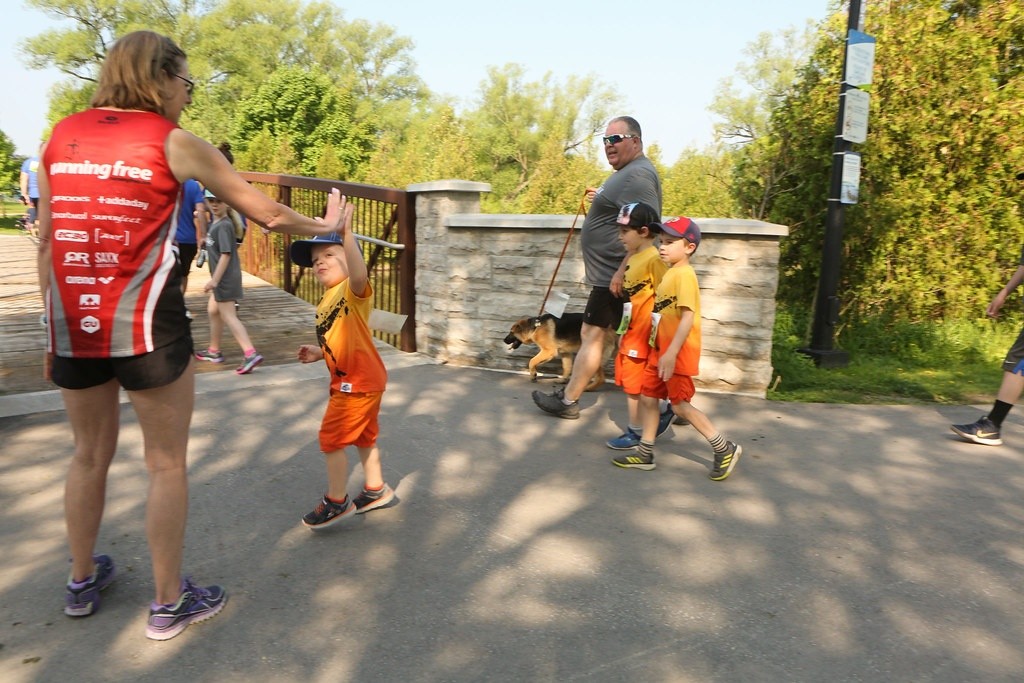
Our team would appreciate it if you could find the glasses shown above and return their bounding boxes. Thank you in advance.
[604,133,640,144]
[165,70,193,96]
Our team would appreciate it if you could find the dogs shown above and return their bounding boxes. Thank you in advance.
[504,312,620,392]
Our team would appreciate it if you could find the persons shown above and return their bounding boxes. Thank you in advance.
[24,200,36,238]
[202,141,246,310]
[297,202,394,528]
[605,203,678,450]
[532,116,690,425]
[950,172,1024,444]
[196,188,264,374]
[611,215,742,480]
[176,179,207,322]
[37,32,345,641]
[20,142,47,224]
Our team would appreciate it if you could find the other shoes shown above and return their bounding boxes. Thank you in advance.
[235,300,239,309]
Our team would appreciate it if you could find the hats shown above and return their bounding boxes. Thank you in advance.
[202,188,216,199]
[289,232,342,267]
[651,215,700,255]
[605,202,661,233]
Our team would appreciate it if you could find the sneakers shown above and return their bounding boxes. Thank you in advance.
[145,578,228,640]
[604,433,642,450]
[351,482,399,514]
[533,390,580,420]
[195,350,223,363]
[707,440,741,480]
[951,415,1003,445]
[609,451,656,470]
[302,493,357,529]
[64,554,116,616]
[236,351,263,375]
[673,412,689,424]
[655,402,675,436]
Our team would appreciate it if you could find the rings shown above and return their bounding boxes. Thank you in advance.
[339,207,344,210]
